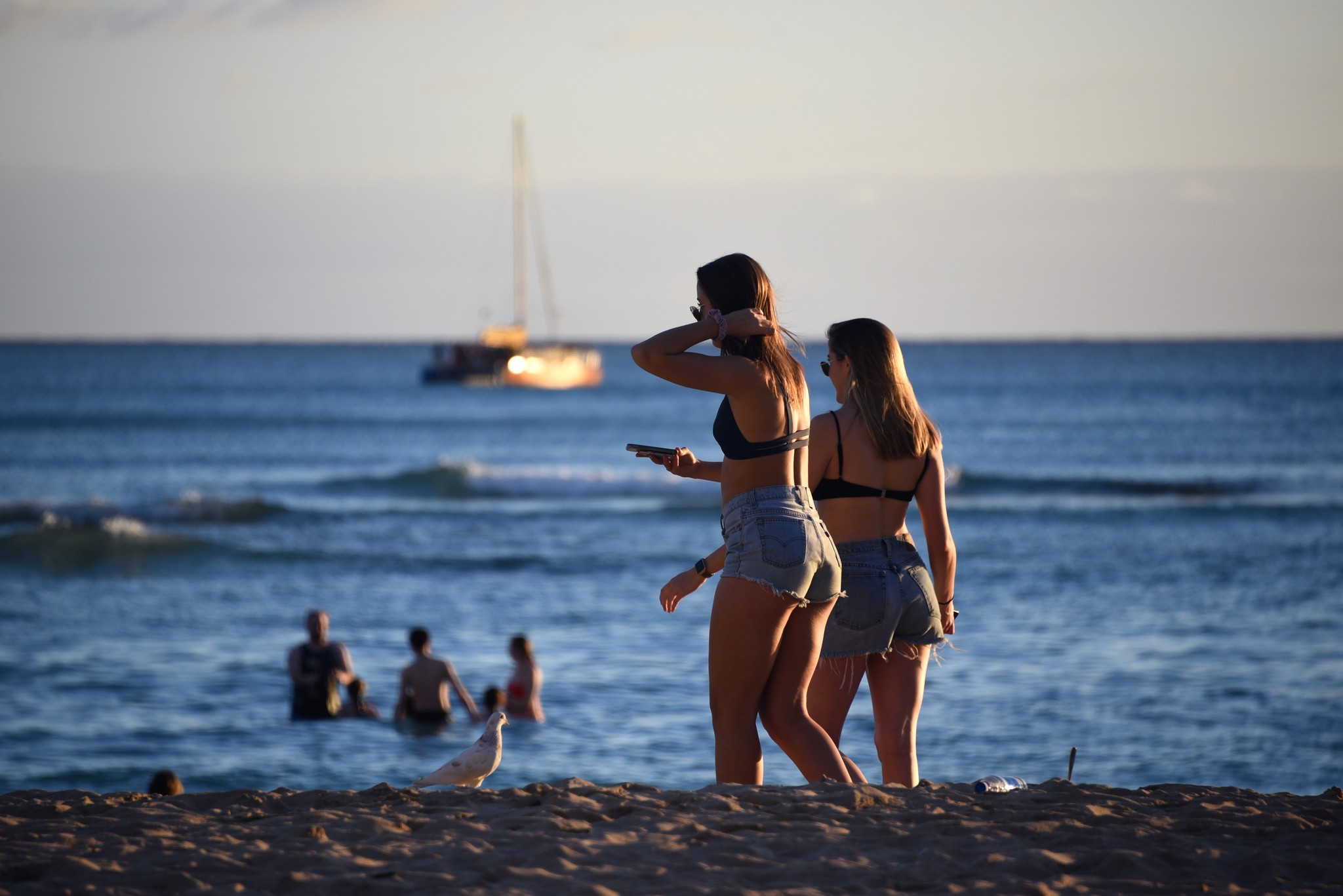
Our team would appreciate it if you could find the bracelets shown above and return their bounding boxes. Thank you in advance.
[705,308,727,343]
[937,594,954,605]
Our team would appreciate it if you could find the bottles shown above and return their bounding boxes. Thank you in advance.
[975,775,1028,795]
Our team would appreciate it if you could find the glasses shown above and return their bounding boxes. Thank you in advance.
[689,300,713,322]
[819,356,844,377]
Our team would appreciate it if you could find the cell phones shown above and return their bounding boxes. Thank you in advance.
[626,442,678,457]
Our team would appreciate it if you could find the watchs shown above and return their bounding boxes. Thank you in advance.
[694,558,716,578]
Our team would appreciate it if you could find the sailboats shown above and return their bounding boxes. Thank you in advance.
[421,108,602,392]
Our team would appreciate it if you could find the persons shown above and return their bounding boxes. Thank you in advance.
[334,677,380,721]
[658,317,958,790]
[394,625,483,726]
[504,634,546,725]
[285,609,356,722]
[147,768,185,798]
[478,686,508,723]
[633,250,856,794]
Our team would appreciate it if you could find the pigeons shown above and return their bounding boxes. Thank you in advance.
[411,713,507,791]
